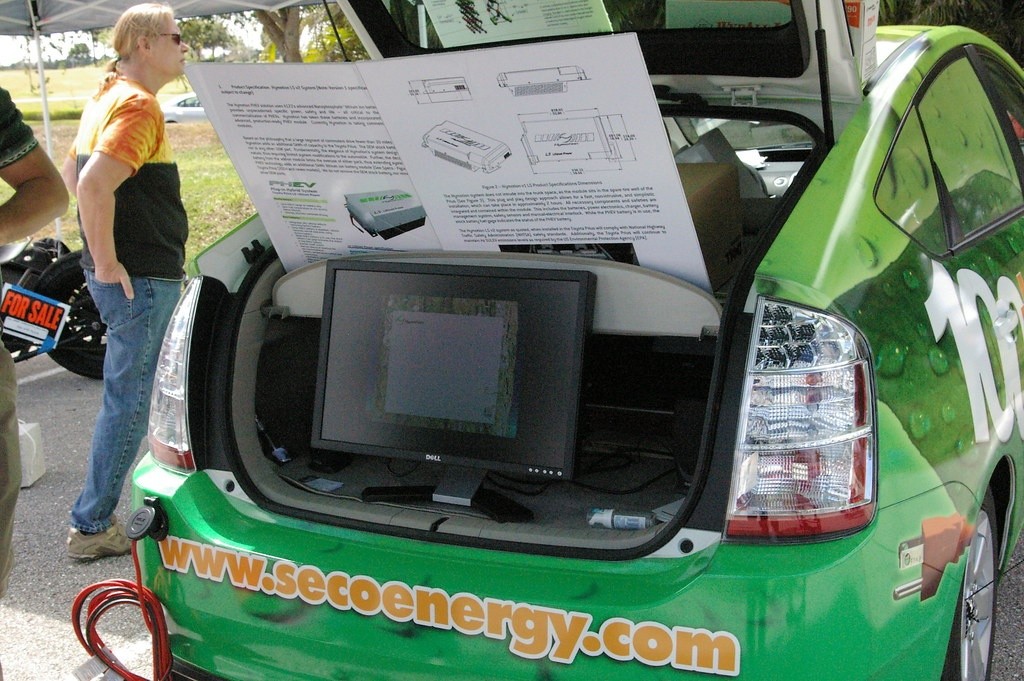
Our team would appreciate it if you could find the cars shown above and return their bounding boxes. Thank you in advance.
[123,0,1024,681]
[158,90,209,124]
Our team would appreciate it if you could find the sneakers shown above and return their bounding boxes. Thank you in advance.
[67,513,131,560]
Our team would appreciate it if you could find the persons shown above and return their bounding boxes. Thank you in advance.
[64,4,188,559]
[0,3,69,599]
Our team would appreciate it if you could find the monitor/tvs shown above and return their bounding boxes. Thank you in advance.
[312,260,598,520]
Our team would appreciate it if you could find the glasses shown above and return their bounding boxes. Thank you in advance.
[160,33,181,45]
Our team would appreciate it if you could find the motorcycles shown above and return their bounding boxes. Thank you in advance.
[0,235,109,382]
